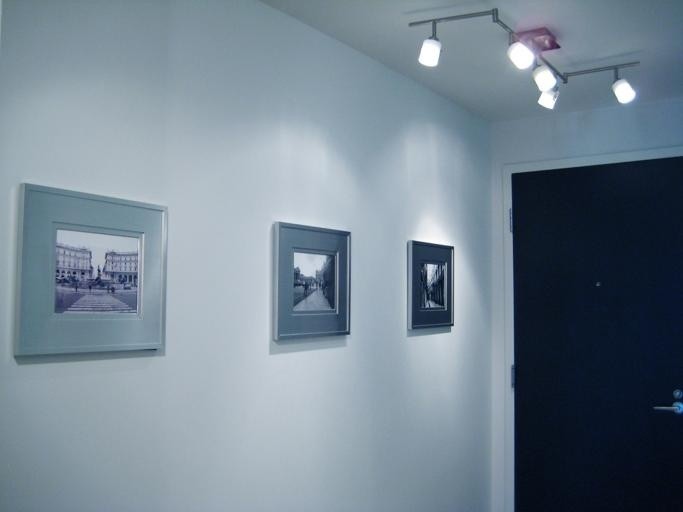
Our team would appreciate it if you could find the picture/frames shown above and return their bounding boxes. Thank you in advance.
[407,239,454,331]
[15,182,167,361]
[273,222,351,338]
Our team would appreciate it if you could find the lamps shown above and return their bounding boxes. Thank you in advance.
[410,8,639,109]
